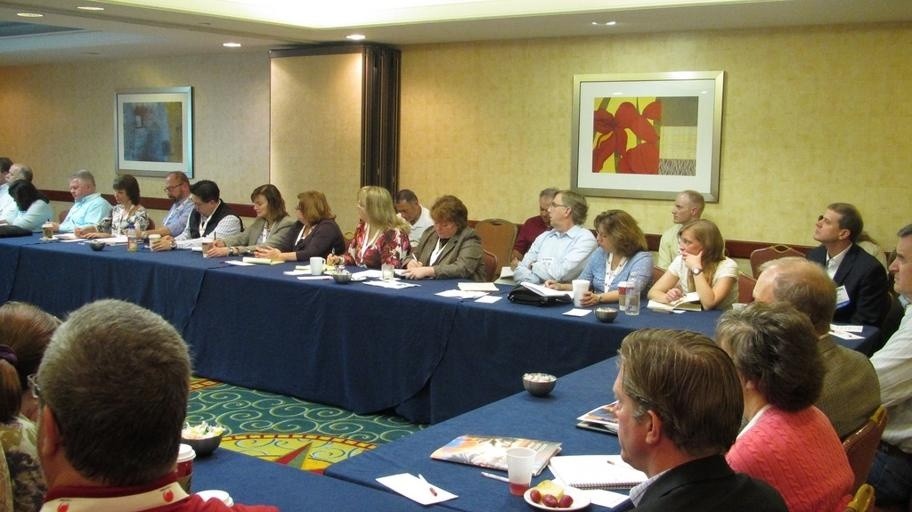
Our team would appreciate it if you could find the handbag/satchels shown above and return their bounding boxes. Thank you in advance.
[507,285,571,307]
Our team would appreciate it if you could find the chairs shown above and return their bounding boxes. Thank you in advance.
[751,244,807,277]
[736,269,758,304]
[474,219,518,280]
[842,405,888,488]
[650,262,668,284]
[342,233,353,252]
[147,216,157,232]
[844,483,877,512]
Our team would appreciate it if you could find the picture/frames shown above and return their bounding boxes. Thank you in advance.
[569,68,726,204]
[113,85,194,181]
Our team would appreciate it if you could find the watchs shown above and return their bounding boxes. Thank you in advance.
[599,296,602,303]
[228,247,233,258]
[689,268,703,274]
[170,240,176,249]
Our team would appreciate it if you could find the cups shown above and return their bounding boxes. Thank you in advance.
[149,234,161,251]
[128,231,137,250]
[617,281,634,310]
[198,490,234,508]
[177,444,196,494]
[382,264,394,283]
[572,280,591,307]
[310,258,326,275]
[202,240,213,257]
[506,447,537,496]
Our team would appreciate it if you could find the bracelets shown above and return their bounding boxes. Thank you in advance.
[235,246,240,256]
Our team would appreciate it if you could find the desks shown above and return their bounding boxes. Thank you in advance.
[189,443,459,512]
[0,232,878,423]
[322,350,651,512]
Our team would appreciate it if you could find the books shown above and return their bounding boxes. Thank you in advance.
[243,257,285,265]
[96,238,129,247]
[395,268,433,280]
[647,290,702,313]
[520,282,574,301]
[429,434,561,476]
[546,454,649,490]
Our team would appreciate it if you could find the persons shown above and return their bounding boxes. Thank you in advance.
[657,190,705,270]
[0,302,59,512]
[401,195,487,283]
[395,189,434,249]
[0,164,33,225]
[511,189,558,270]
[327,186,412,270]
[42,170,112,234]
[8,178,54,233]
[543,209,653,306]
[805,203,890,327]
[152,180,244,252]
[648,220,739,311]
[35,300,279,512]
[610,330,788,512]
[139,171,193,240]
[868,222,912,511]
[716,301,854,511]
[74,174,148,239]
[207,184,296,258]
[0,157,12,213]
[514,190,598,286]
[752,256,881,441]
[254,191,345,262]
[854,234,888,277]
[868,249,908,358]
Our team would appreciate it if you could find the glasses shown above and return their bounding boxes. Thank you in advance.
[26,373,63,434]
[163,183,183,193]
[551,200,567,208]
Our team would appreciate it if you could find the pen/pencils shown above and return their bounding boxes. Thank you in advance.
[213,231,216,240]
[332,248,335,267]
[481,471,509,483]
[71,218,78,228]
[416,472,437,497]
[412,252,418,262]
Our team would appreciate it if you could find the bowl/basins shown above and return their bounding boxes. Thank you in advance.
[182,427,225,452]
[595,308,618,321]
[333,273,353,282]
[90,243,105,250]
[523,373,557,395]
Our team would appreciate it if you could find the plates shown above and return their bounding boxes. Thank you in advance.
[524,486,591,510]
